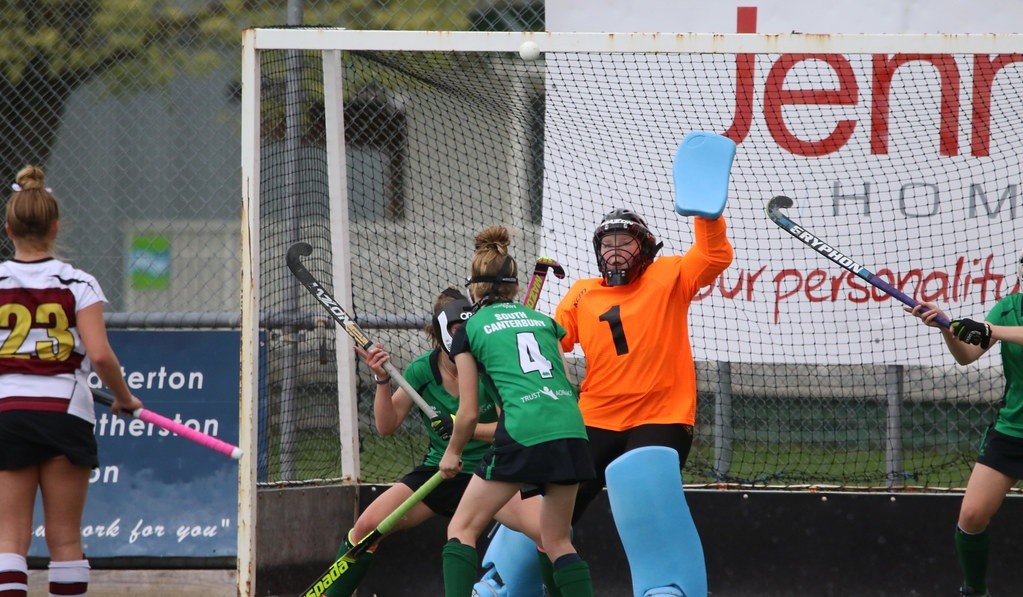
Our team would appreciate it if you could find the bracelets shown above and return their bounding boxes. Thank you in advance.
[375,374,390,384]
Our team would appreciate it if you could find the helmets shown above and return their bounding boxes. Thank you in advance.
[592,209,664,286]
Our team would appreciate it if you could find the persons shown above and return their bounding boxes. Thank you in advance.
[903,255,1023,597]
[439,225,595,597]
[0,165,143,597]
[474,208,733,597]
[319,287,574,597]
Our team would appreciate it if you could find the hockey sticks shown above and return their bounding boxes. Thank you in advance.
[764,194,983,348]
[88,384,245,461]
[520,255,568,310]
[300,457,464,597]
[281,239,448,436]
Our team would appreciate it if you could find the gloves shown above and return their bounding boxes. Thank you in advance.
[951,318,992,350]
[431,413,472,444]
